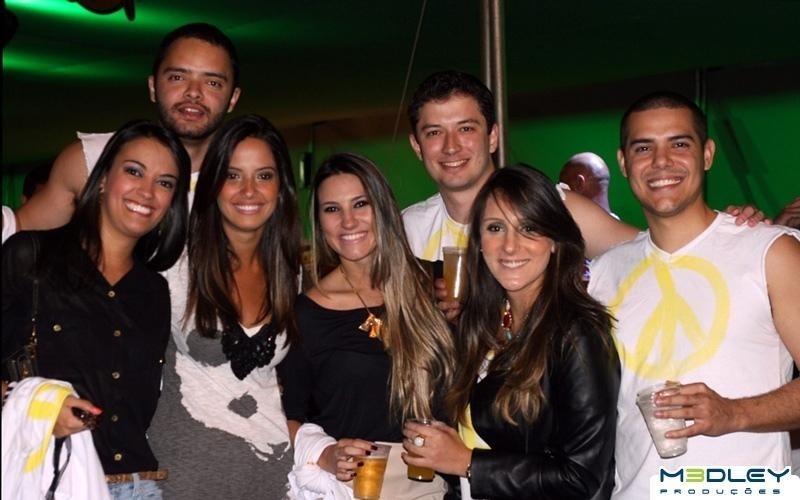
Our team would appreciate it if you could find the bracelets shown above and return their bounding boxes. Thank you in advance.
[466,463,472,485]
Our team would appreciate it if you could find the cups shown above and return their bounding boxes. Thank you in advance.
[404,417,439,482]
[350,441,392,499]
[441,246,471,305]
[634,382,689,459]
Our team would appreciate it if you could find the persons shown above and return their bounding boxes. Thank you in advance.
[151,115,313,498]
[772,191,800,228]
[401,159,622,499]
[1,114,194,498]
[557,150,622,221]
[394,67,775,331]
[277,150,453,500]
[1,21,242,251]
[21,161,51,206]
[578,82,800,500]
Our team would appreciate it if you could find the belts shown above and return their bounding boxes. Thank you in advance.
[105,469,168,483]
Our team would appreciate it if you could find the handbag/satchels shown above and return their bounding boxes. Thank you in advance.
[6,345,39,382]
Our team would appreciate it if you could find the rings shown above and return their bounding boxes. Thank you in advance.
[414,433,427,448]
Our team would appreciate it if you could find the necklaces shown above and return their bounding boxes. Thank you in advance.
[338,264,389,342]
[499,297,518,348]
